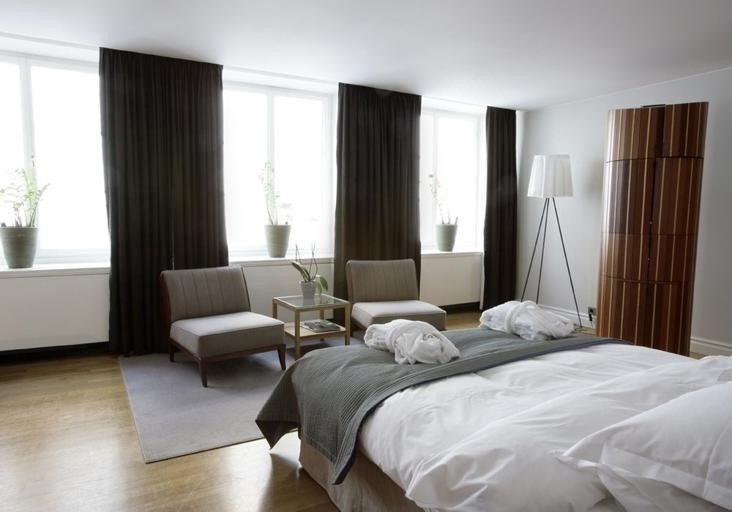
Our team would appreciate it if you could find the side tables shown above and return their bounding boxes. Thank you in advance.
[272,293,351,360]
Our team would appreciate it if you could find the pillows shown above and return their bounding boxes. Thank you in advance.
[551,380,732,511]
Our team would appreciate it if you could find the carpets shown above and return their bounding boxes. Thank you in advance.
[117,330,365,465]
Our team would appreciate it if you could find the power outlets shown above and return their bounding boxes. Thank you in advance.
[588,307,596,316]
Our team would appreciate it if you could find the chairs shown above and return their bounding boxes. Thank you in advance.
[345,258,446,338]
[159,265,286,388]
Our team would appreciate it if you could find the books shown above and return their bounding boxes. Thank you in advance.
[299,319,340,332]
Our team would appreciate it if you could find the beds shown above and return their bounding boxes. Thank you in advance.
[253,325,732,512]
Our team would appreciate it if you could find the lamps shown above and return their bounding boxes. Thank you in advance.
[520,154,584,328]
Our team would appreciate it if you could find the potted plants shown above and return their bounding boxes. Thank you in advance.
[428,176,458,252]
[259,161,292,258]
[0,167,52,268]
[291,260,328,298]
